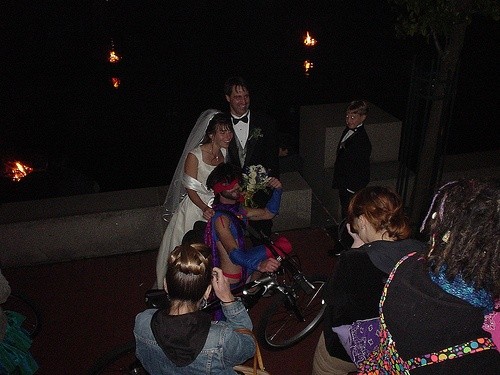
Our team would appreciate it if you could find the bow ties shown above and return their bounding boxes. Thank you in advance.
[348,127,358,132]
[231,111,249,125]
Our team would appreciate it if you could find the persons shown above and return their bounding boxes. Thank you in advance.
[134,73,294,375]
[327,96,372,256]
[312,171,500,375]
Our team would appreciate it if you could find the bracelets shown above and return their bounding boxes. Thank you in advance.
[222,298,239,304]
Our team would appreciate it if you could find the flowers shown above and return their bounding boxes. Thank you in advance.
[239,160,272,208]
[249,119,264,141]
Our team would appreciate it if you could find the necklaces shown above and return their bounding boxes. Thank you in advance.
[209,149,221,162]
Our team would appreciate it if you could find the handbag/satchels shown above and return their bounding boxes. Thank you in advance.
[233,328,269,375]
[358,315,410,375]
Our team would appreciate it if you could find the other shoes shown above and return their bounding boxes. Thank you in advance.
[327,247,344,256]
[247,287,273,298]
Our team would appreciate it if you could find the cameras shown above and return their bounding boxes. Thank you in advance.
[211,272,218,282]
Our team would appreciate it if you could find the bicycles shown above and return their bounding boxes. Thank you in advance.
[81,212,331,375]
